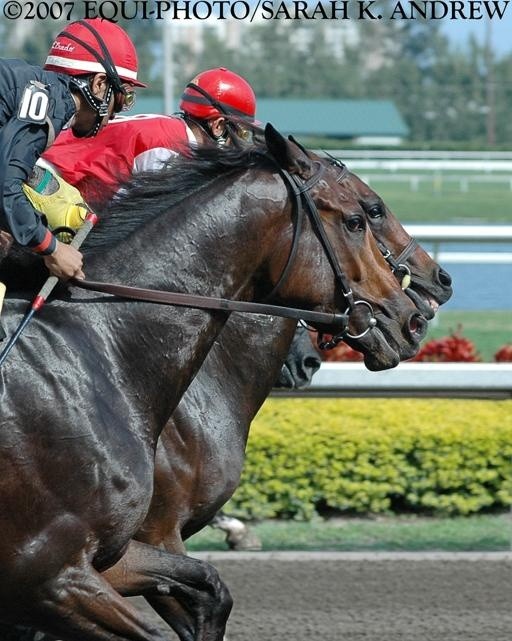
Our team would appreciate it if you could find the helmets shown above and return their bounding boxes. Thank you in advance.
[43,17,148,90]
[180,67,262,128]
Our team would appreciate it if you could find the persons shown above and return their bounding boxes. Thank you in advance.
[20,67,267,248]
[1,17,148,283]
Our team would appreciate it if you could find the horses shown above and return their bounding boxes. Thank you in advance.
[1,120,454,641]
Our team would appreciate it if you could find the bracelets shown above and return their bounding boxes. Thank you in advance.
[30,226,58,256]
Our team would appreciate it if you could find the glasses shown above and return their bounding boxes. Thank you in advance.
[186,83,251,140]
[56,19,136,113]
